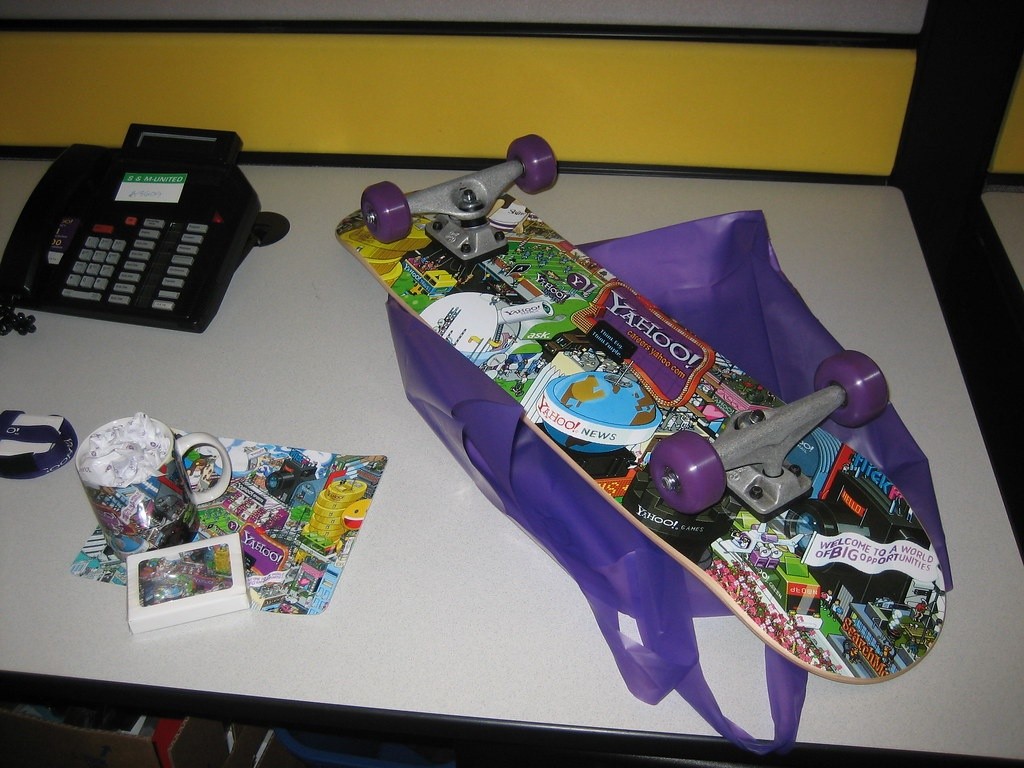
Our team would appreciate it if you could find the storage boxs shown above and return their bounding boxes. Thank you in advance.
[0,696,456,768]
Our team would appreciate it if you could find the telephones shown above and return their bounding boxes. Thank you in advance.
[1,119,263,339]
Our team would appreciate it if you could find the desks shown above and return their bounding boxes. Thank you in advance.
[0,157,1024,768]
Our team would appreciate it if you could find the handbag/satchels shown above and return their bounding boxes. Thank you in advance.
[385,211,954,757]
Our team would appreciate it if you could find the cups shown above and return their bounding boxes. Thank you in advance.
[75,413,233,564]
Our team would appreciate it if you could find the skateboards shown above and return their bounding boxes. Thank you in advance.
[335,134,948,685]
